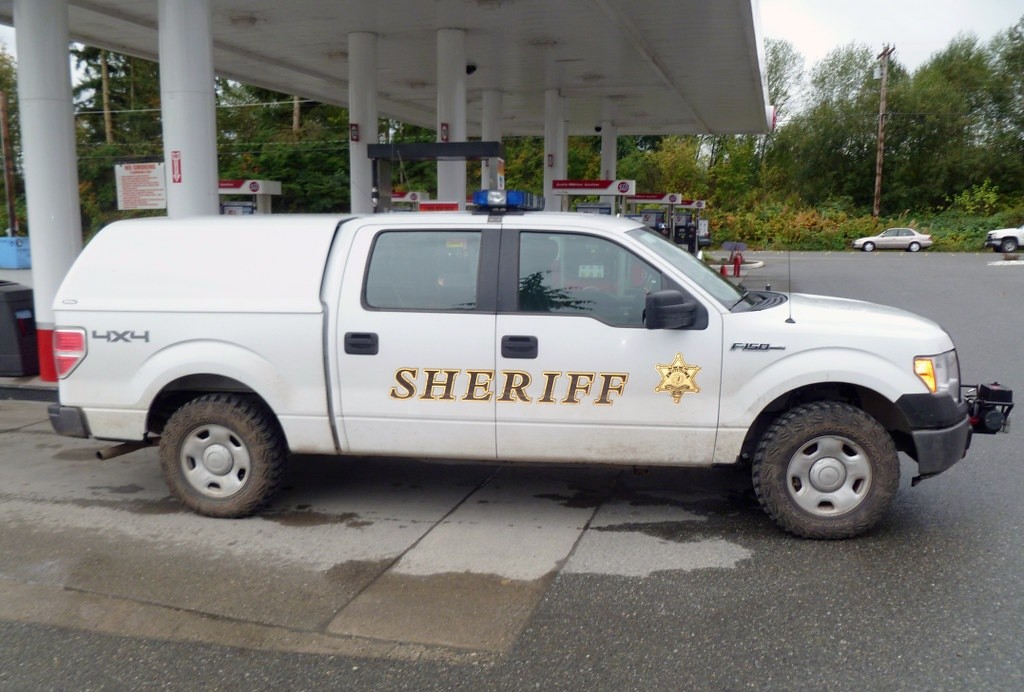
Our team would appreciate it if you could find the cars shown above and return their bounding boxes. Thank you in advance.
[697,230,712,249]
[851,228,934,253]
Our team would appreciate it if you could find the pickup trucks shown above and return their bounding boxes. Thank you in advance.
[47,188,1014,543]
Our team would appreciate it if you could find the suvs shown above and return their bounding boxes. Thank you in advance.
[985,223,1024,252]
